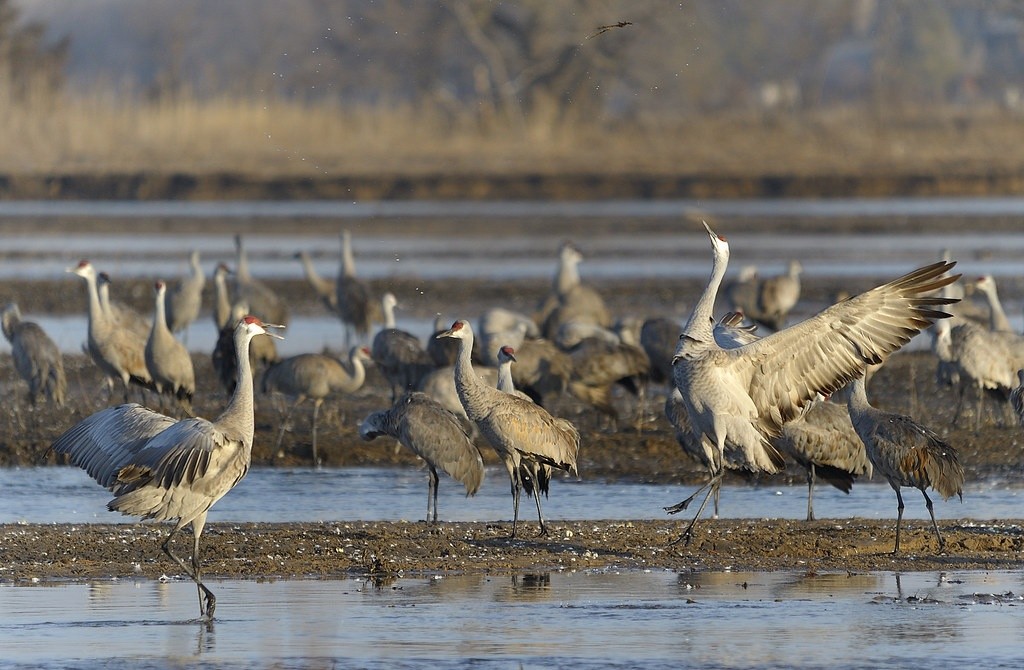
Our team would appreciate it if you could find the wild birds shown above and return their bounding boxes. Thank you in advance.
[373,293,437,404]
[728,259,805,331]
[37,314,286,623]
[361,392,484,526]
[166,244,205,335]
[777,398,875,522]
[0,295,67,408]
[436,319,581,543]
[212,232,290,333]
[295,227,383,353]
[496,346,533,520]
[667,380,743,519]
[259,343,374,469]
[426,240,685,437]
[849,363,964,557]
[663,219,962,550]
[211,263,278,420]
[929,249,1024,435]
[145,279,195,417]
[65,260,162,407]
